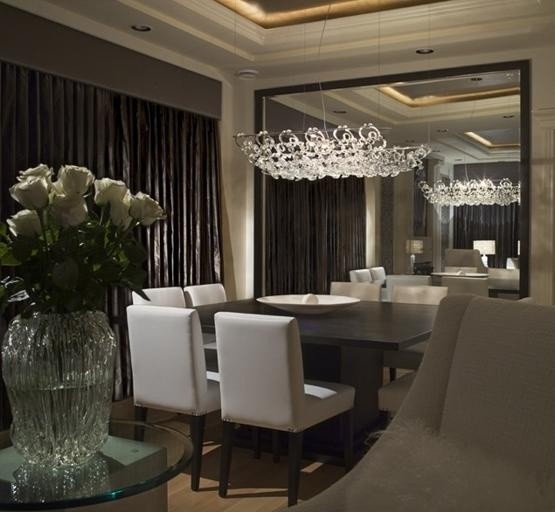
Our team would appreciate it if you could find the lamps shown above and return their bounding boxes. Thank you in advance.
[417,76,521,208]
[229,2,433,186]
[472,240,497,268]
[405,239,425,273]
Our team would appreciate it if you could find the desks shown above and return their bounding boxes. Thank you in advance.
[0,417,194,510]
[187,297,440,458]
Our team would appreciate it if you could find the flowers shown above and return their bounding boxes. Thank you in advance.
[0,162,168,387]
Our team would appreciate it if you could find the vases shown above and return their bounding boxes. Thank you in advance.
[1,309,121,465]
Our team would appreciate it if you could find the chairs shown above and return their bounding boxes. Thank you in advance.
[443,248,488,273]
[131,286,186,309]
[430,272,491,296]
[182,282,227,306]
[213,310,358,507]
[391,283,449,304]
[369,266,387,280]
[329,280,383,302]
[125,304,262,493]
[274,294,555,511]
[348,269,384,285]
[385,274,434,302]
[377,294,535,436]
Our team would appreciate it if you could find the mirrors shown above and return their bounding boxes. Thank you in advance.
[251,56,534,303]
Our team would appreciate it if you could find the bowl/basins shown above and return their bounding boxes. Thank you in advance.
[255,294,360,316]
